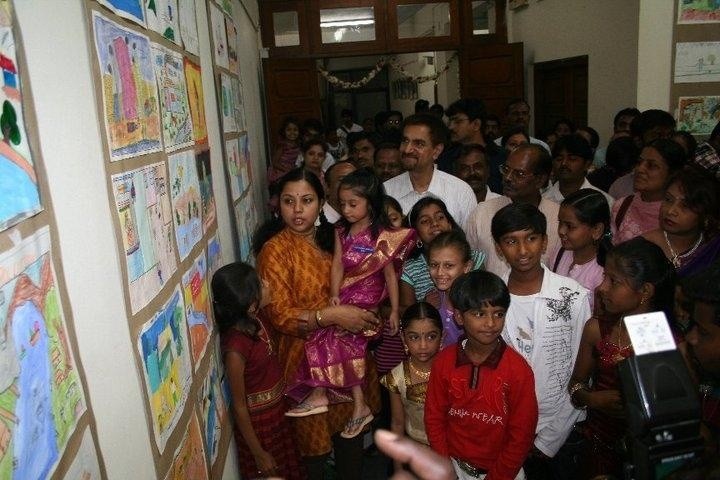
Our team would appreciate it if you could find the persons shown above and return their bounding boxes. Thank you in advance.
[169,377,179,407]
[106,32,144,125]
[210,101,720,480]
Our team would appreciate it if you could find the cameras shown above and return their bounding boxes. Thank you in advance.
[617,311,720,480]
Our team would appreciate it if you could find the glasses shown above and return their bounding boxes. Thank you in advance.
[499,164,533,179]
